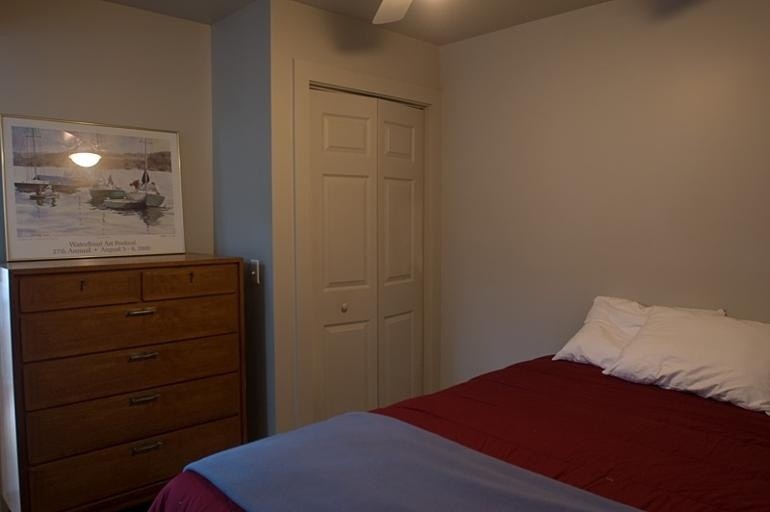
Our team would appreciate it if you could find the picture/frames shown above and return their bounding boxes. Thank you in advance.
[0,113,186,264]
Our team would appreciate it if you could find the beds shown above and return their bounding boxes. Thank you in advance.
[150,327,770,511]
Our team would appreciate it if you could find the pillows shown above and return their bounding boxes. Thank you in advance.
[551,295,769,416]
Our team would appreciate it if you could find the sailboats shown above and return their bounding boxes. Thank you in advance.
[14,127,165,211]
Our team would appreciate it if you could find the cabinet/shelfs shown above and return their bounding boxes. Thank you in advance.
[0,253,250,511]
